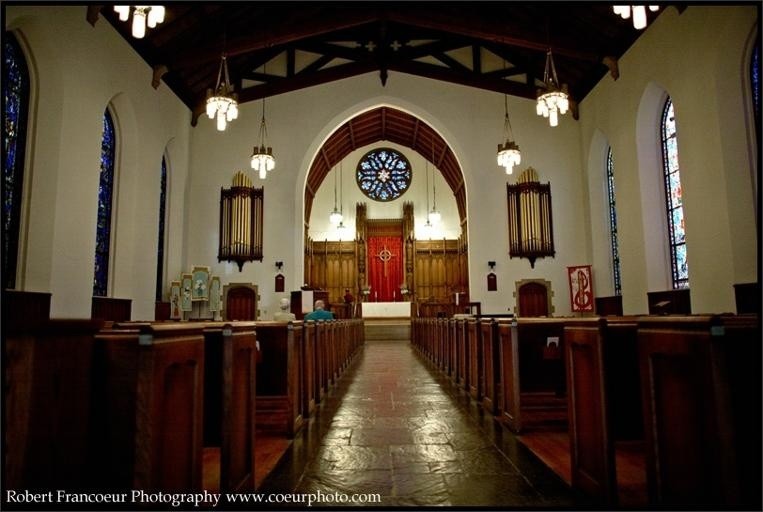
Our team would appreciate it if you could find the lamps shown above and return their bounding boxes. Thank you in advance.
[114,6,165,39]
[330,161,346,234]
[506,166,556,269]
[217,171,264,272]
[613,5,659,31]
[424,136,441,235]
[496,38,521,175]
[250,46,275,179]
[206,57,238,132]
[536,48,569,126]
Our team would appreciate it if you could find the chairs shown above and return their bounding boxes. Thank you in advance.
[410,315,763,511]
[0,320,365,512]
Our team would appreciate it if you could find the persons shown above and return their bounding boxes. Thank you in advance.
[272,298,296,321]
[343,287,355,319]
[303,298,331,321]
